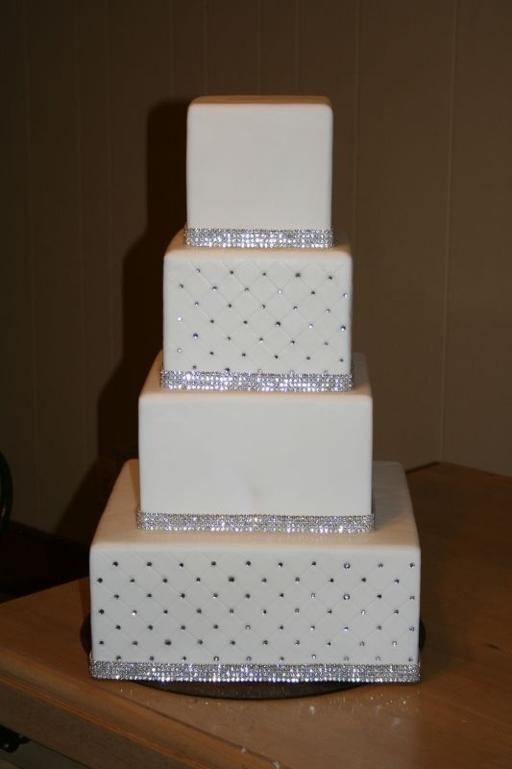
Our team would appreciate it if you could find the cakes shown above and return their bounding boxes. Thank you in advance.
[90,95,425,685]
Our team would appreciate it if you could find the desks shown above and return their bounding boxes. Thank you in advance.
[1,462,512,769]
